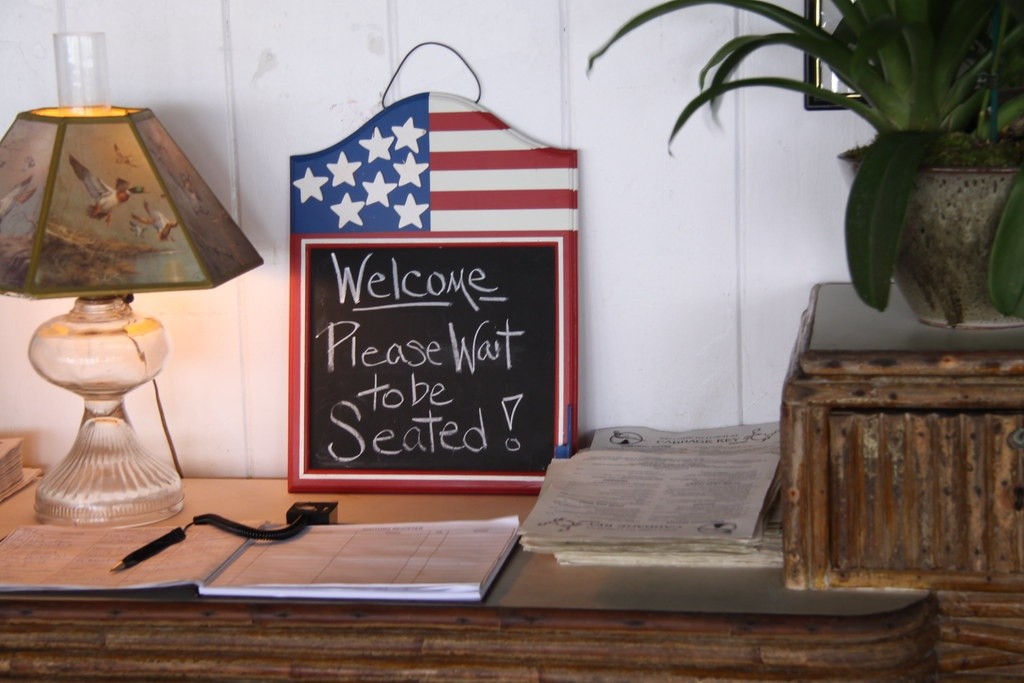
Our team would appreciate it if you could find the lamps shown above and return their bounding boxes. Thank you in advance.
[0,31,264,531]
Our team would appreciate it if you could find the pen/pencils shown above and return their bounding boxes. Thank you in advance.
[109,527,186,572]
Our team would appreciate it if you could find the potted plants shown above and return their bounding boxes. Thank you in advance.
[584,0,1024,330]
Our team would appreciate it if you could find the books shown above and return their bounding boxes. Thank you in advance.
[0,514,519,602]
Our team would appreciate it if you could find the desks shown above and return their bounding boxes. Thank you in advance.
[0,282,1024,683]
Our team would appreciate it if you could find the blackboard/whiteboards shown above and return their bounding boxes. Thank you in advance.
[288,232,578,498]
